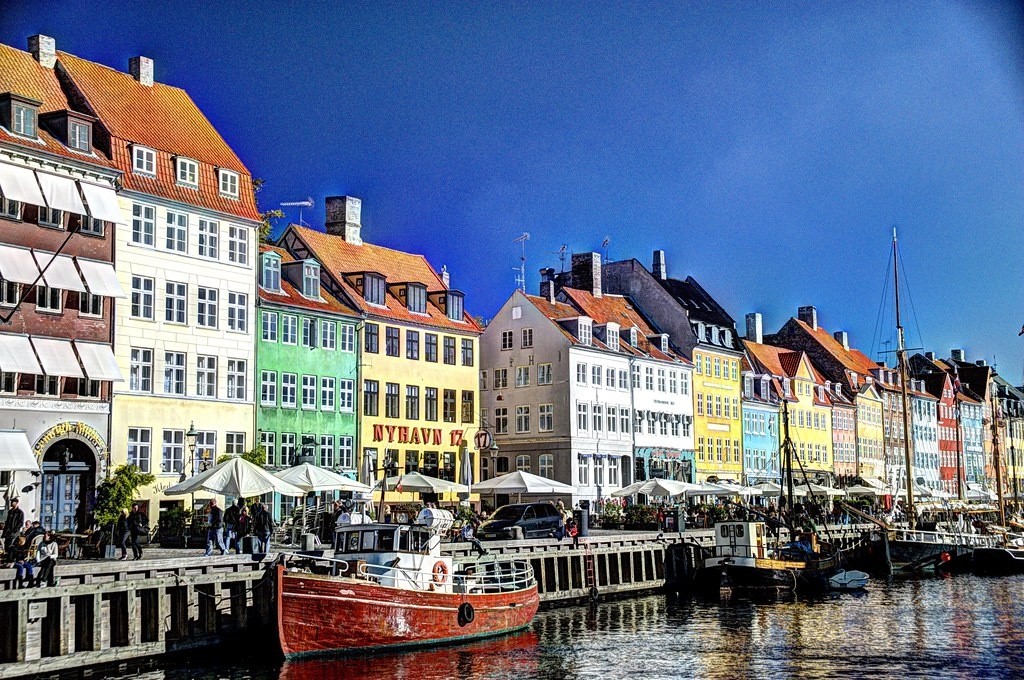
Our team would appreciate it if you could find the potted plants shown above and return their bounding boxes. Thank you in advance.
[81,463,157,558]
[623,503,662,531]
[157,506,196,549]
[601,501,624,530]
[187,522,226,549]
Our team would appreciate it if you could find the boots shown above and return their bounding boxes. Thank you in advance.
[18,577,26,589]
[28,574,36,588]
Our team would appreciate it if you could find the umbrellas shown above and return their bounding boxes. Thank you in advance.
[362,449,375,496]
[459,445,472,502]
[461,471,577,502]
[370,471,470,507]
[269,461,371,516]
[611,476,1024,503]
[162,454,307,510]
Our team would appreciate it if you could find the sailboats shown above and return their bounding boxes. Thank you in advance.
[829,222,1024,580]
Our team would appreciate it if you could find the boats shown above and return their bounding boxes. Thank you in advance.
[696,396,841,603]
[827,569,871,590]
[274,453,541,662]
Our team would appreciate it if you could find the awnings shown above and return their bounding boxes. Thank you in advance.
[0,159,129,226]
[0,428,41,473]
[0,242,128,299]
[0,331,127,383]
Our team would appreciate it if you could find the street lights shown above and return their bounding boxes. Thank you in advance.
[184,419,200,529]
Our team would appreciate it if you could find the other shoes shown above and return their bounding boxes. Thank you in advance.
[121,556,128,561]
[205,551,212,557]
[221,549,229,555]
[136,553,144,560]
[132,554,139,561]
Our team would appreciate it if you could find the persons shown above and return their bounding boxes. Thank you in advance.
[115,502,150,561]
[645,497,1024,535]
[462,518,489,556]
[548,498,579,550]
[332,499,358,521]
[0,497,58,589]
[384,500,489,543]
[203,495,277,556]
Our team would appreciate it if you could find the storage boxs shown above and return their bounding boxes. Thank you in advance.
[453,580,476,593]
[337,560,367,574]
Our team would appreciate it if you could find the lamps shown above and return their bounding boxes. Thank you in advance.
[496,383,505,401]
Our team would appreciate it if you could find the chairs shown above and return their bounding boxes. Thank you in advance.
[24,534,44,561]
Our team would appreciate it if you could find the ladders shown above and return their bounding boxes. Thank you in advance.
[586,544,594,592]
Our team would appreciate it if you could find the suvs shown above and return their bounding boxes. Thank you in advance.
[475,502,563,540]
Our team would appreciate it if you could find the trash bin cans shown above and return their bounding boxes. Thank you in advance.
[573,510,589,537]
[243,536,260,566]
[862,504,871,523]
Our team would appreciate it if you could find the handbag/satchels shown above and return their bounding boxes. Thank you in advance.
[136,525,150,537]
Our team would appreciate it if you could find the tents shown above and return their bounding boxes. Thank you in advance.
[862,477,888,489]
[968,484,995,496]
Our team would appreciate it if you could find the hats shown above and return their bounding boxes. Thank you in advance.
[11,498,18,502]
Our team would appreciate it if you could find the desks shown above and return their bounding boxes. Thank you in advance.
[56,534,88,559]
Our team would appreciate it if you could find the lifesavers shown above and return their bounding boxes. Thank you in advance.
[459,603,474,623]
[433,561,449,586]
[590,587,599,600]
